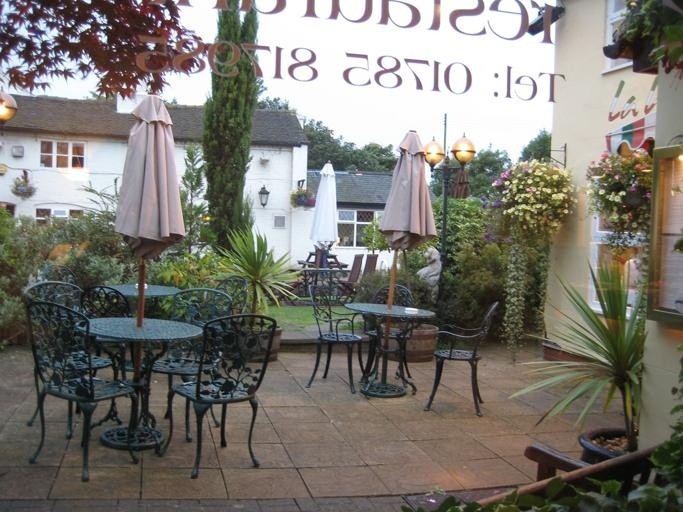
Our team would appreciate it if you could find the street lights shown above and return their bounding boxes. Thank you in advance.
[422,131,476,339]
[0,84,17,136]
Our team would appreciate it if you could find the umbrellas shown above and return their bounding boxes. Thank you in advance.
[98,94,187,450]
[357,127,440,399]
[305,157,337,305]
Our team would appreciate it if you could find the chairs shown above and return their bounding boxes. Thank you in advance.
[20,273,248,465]
[401,438,670,510]
[305,284,370,395]
[159,314,277,478]
[424,301,499,417]
[359,284,417,378]
[27,301,139,482]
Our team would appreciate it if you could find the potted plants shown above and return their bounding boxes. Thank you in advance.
[503,240,650,465]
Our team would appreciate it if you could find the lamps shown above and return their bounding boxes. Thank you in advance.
[256,184,270,209]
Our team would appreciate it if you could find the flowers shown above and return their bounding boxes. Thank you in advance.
[8,169,40,203]
[477,147,653,367]
[287,187,316,212]
